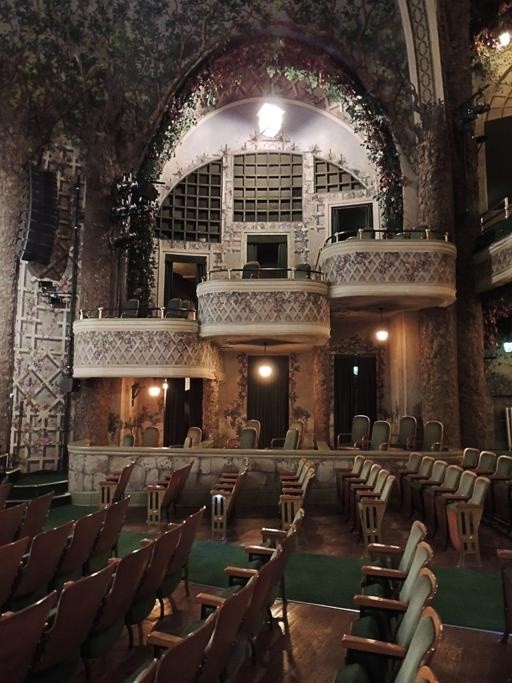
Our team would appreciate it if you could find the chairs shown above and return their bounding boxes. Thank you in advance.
[294,264,311,279]
[241,261,262,279]
[346,225,430,240]
[147,298,191,318]
[105,299,139,318]
[0,414,512,683]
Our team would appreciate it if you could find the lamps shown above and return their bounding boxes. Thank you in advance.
[376,307,388,341]
[149,377,160,397]
[256,101,286,138]
[258,342,271,377]
